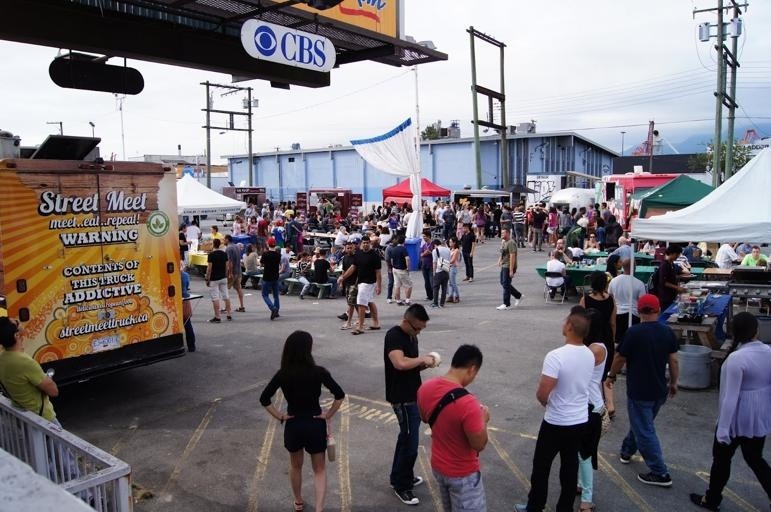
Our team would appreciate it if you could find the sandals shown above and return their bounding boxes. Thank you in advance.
[577,503,597,511]
[292,499,305,512]
[576,486,582,496]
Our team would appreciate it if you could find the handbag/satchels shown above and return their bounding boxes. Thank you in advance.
[599,408,611,438]
[436,257,450,272]
[558,227,566,234]
[547,228,553,235]
[326,417,335,462]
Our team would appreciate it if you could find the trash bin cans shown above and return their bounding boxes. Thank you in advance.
[232,235,251,259]
[675,344,713,390]
[404,237,422,271]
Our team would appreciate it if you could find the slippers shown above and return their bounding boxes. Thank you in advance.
[351,329,364,335]
[365,326,380,330]
[341,324,352,330]
[354,321,366,326]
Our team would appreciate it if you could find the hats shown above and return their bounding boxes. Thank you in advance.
[268,238,276,246]
[314,247,320,253]
[638,294,660,313]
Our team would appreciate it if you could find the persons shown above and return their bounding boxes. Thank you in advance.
[688,310,771,512]
[0,315,109,507]
[579,269,620,419]
[416,340,492,511]
[511,304,598,511]
[604,292,684,489]
[576,307,611,512]
[174,197,771,338]
[256,328,346,512]
[383,300,442,507]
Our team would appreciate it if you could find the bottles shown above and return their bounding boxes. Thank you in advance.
[326,434,336,462]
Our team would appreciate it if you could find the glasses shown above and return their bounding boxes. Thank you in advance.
[353,242,358,245]
[408,320,421,331]
[590,236,594,238]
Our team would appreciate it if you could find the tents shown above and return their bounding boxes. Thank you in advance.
[629,146,770,328]
[636,174,717,219]
[176,172,246,220]
[382,176,450,207]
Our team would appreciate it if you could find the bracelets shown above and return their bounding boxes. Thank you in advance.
[281,413,286,424]
[607,370,618,379]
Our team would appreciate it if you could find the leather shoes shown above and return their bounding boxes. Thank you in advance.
[689,493,721,511]
[462,277,469,281]
[469,277,473,282]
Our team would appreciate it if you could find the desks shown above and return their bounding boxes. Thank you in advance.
[187,223,439,301]
[536,240,767,387]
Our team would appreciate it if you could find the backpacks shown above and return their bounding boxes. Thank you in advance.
[647,268,657,293]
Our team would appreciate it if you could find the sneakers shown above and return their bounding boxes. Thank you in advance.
[515,294,526,306]
[514,503,547,512]
[386,299,392,304]
[227,315,232,320]
[395,486,420,504]
[271,308,279,320]
[365,312,371,318]
[209,317,221,323]
[329,295,337,299]
[619,452,632,464]
[298,295,304,299]
[394,297,405,302]
[405,301,416,305]
[390,475,423,489]
[497,303,513,310]
[427,303,439,309]
[221,308,227,313]
[236,307,245,312]
[337,313,348,321]
[638,471,672,486]
[279,289,286,295]
[396,300,405,306]
